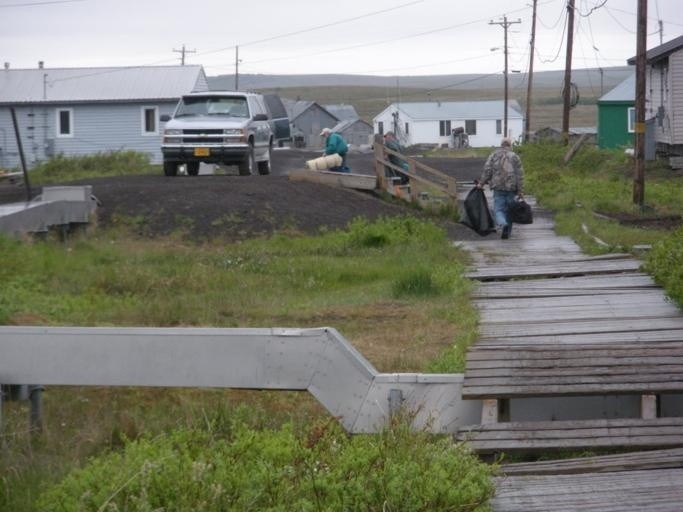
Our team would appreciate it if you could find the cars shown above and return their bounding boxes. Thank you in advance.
[161,92,273,175]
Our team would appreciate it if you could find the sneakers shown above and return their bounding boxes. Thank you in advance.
[501,225,509,239]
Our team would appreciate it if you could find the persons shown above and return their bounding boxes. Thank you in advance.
[320,127,351,173]
[475,136,525,239]
[383,130,409,185]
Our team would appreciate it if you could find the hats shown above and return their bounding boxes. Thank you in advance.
[319,128,332,136]
[384,131,395,138]
[501,137,512,144]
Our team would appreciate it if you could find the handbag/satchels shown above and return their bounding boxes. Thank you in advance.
[508,198,533,224]
